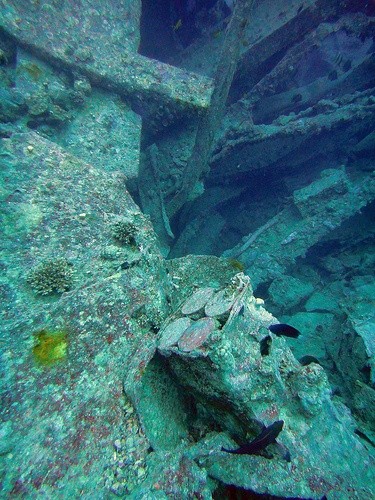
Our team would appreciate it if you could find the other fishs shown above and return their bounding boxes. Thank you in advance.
[220,420,284,455]
[266,324,303,341]
